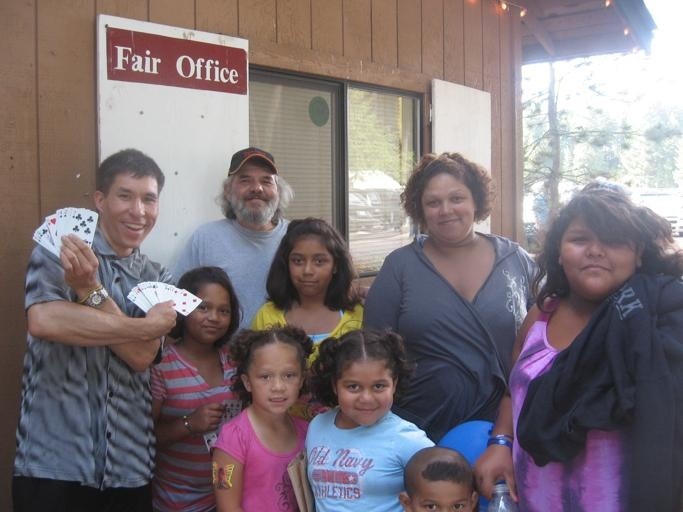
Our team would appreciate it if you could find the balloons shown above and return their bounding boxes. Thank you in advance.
[434,419,494,510]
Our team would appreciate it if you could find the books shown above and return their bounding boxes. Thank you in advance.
[288,444,315,511]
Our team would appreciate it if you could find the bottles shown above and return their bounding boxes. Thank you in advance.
[488,480,519,512]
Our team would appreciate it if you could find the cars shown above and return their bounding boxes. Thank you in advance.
[348,192,384,234]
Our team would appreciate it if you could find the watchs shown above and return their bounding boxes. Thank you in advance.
[76,281,112,313]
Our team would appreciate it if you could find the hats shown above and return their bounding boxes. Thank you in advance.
[228,145,277,178]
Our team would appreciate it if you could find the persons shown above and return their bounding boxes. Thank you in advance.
[212,323,315,511]
[11,147,175,509]
[304,328,435,511]
[164,146,294,332]
[472,182,682,512]
[398,447,478,512]
[364,151,543,446]
[150,264,251,511]
[254,216,363,428]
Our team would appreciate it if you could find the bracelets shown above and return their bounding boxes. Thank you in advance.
[487,432,515,452]
[180,413,198,437]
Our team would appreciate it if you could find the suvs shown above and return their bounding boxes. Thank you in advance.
[350,169,411,233]
[638,186,682,238]
